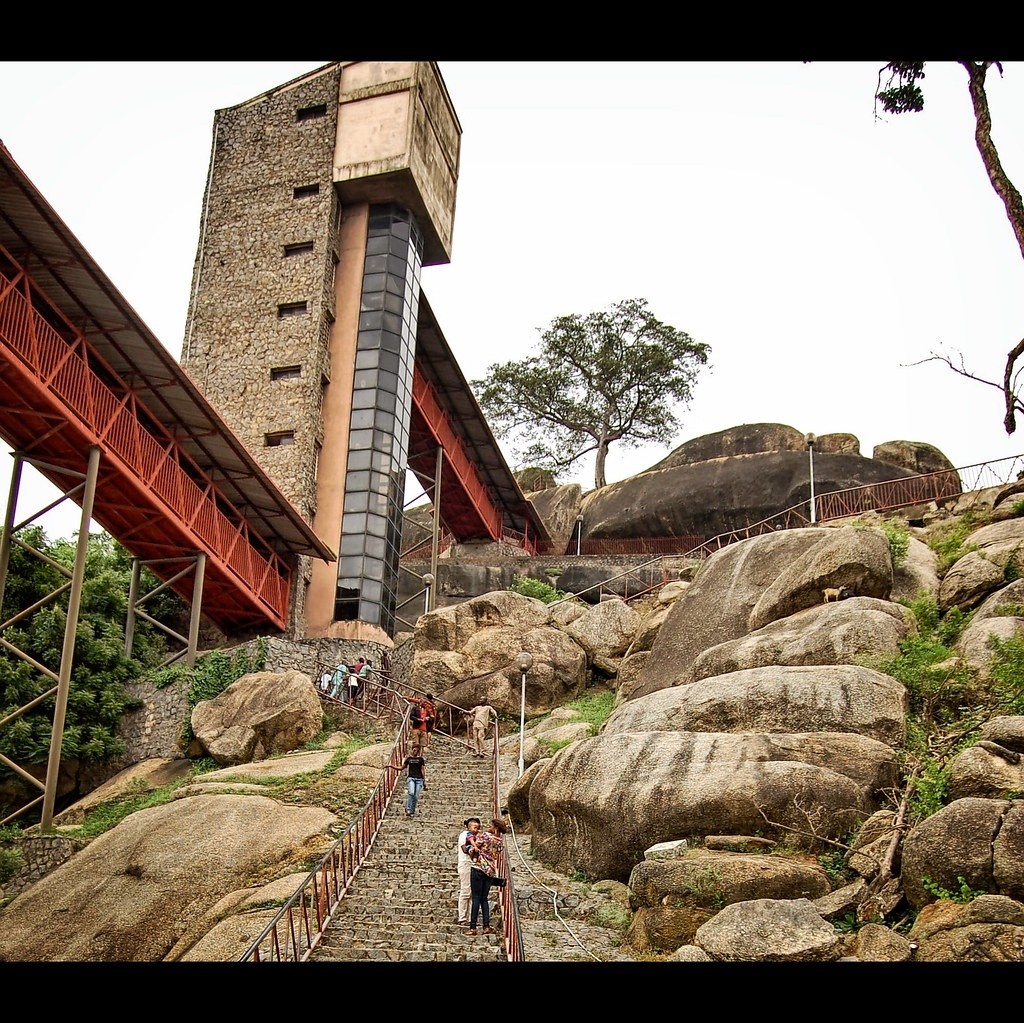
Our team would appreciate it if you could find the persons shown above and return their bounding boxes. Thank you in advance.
[359,660,372,697]
[465,820,481,862]
[464,819,507,935]
[350,657,364,674]
[459,696,498,758]
[347,669,359,705]
[401,694,437,745]
[383,744,426,816]
[458,818,481,926]
[319,670,333,691]
[380,653,389,685]
[330,664,350,703]
[410,697,434,760]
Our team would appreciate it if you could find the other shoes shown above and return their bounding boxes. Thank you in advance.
[483,926,491,934]
[472,753,484,758]
[464,929,477,935]
[459,920,481,926]
[406,809,414,817]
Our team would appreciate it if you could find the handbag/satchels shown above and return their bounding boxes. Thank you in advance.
[491,876,507,887]
